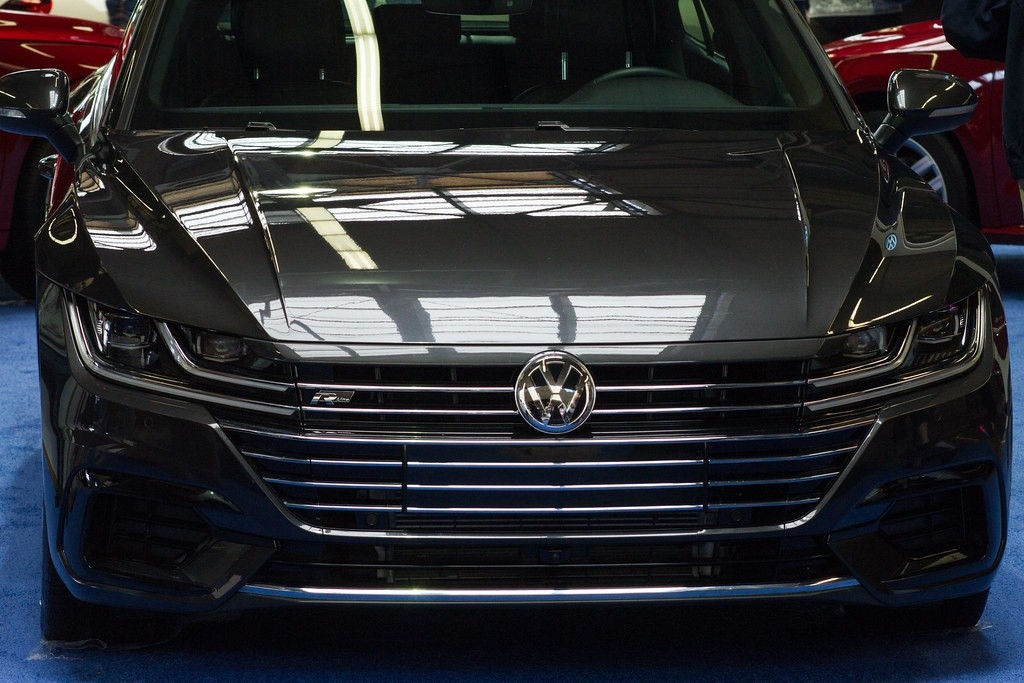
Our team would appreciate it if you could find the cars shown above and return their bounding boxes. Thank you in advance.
[0,0,1013,642]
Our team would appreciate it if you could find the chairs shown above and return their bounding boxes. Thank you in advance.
[197,0,357,106]
[510,0,663,105]
